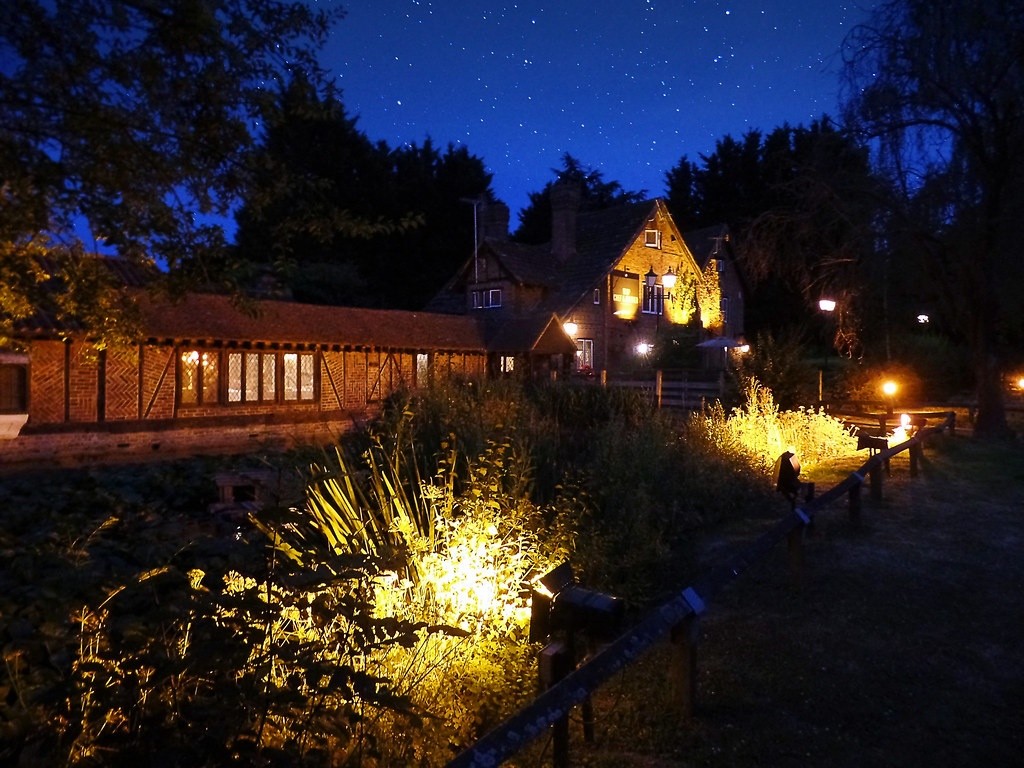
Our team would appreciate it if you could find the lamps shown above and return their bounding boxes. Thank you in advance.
[857,431,888,450]
[530,560,626,643]
[773,451,801,500]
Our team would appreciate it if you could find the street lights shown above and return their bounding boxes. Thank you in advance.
[814,295,837,402]
[643,262,678,336]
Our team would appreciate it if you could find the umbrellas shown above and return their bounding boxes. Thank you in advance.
[695,337,744,371]
